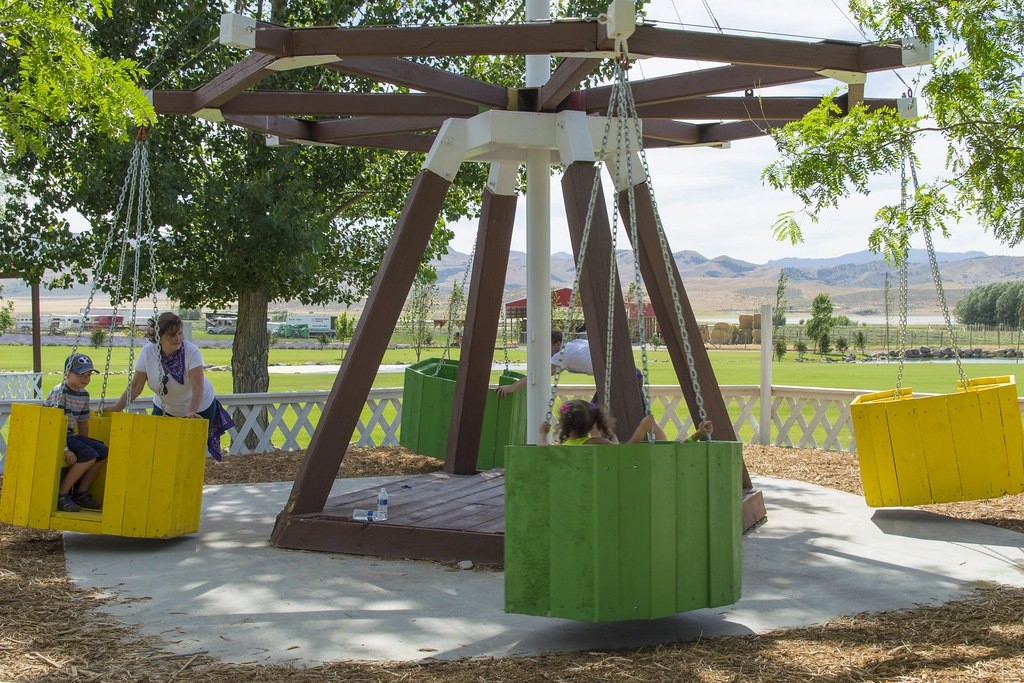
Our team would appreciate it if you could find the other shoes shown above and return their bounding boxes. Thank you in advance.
[57,493,80,512]
[73,491,101,509]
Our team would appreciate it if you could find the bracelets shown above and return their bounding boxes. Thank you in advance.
[687,437,696,443]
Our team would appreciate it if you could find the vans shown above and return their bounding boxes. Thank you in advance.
[16,318,33,330]
[207,317,238,334]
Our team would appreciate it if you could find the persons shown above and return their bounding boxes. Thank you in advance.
[42,354,109,512]
[624,414,713,443]
[493,330,654,417]
[538,398,620,445]
[100,311,217,432]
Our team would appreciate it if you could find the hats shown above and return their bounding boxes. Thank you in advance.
[63,353,101,374]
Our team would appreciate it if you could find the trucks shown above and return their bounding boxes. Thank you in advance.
[271,315,339,338]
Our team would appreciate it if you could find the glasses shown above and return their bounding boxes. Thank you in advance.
[159,375,169,397]
